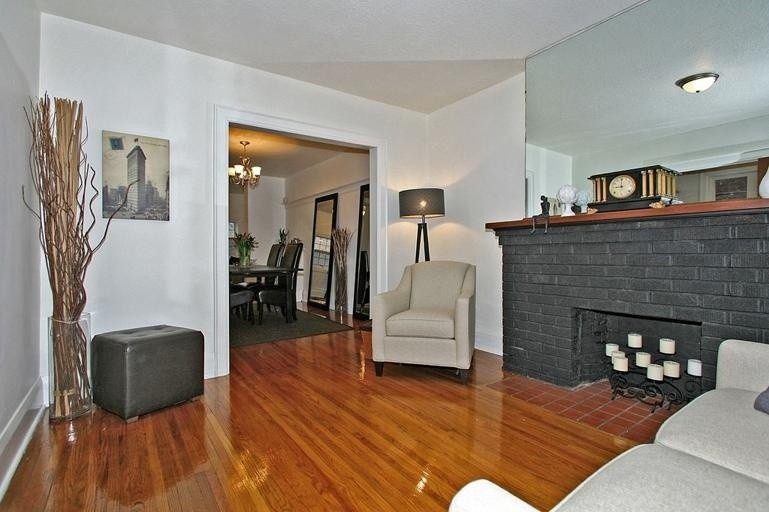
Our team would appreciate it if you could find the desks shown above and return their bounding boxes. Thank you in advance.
[229,264,304,323]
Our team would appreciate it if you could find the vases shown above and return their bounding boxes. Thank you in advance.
[49,313,91,419]
[239,246,250,269]
[333,255,347,314]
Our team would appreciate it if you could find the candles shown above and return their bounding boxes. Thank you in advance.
[605,334,702,381]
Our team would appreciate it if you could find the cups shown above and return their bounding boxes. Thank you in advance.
[249,259,258,266]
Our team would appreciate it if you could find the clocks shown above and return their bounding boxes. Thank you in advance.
[608,174,636,199]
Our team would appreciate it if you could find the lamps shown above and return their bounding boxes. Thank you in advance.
[399,188,444,263]
[675,72,719,95]
[229,141,262,192]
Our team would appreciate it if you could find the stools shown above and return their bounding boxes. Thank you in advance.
[91,324,204,425]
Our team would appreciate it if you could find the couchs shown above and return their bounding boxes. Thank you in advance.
[371,262,476,384]
[449,339,769,512]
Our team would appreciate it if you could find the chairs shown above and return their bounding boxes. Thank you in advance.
[229,285,255,321]
[248,243,303,325]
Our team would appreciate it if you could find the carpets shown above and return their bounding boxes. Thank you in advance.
[230,302,355,347]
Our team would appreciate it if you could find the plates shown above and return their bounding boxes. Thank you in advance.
[235,265,254,269]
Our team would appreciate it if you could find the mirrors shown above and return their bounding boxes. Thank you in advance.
[352,184,369,321]
[307,193,338,311]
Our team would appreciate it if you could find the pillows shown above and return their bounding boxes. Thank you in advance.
[754,386,769,414]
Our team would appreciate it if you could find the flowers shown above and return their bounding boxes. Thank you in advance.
[232,232,259,256]
